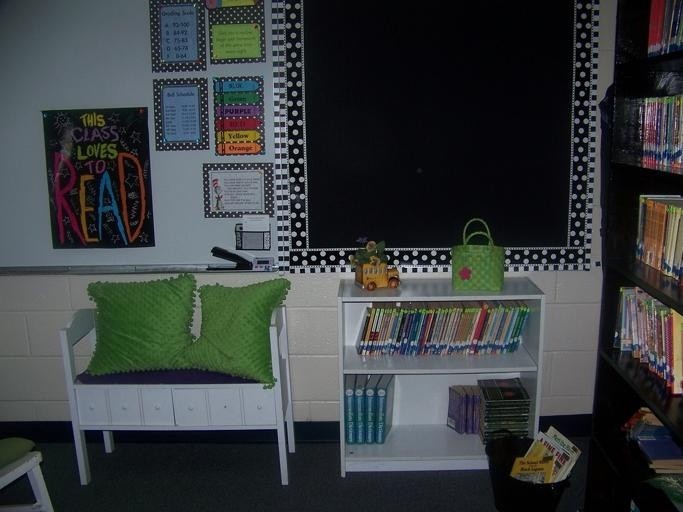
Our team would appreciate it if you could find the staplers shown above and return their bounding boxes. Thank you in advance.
[206,247,253,270]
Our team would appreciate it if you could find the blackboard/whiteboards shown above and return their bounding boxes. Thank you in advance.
[0,0,279,277]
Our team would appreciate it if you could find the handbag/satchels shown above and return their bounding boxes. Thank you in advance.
[452,219,504,291]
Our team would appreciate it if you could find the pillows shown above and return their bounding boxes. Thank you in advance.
[87,276,290,383]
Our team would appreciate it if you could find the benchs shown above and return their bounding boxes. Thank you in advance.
[61,305,297,487]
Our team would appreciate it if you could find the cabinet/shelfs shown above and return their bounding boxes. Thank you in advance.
[589,2,682,510]
[338,295,544,478]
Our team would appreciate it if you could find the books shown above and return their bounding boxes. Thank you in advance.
[344,374,356,446]
[375,374,395,445]
[352,299,531,358]
[354,374,367,446]
[615,0,683,477]
[363,374,382,444]
[507,425,582,484]
[443,376,530,445]
